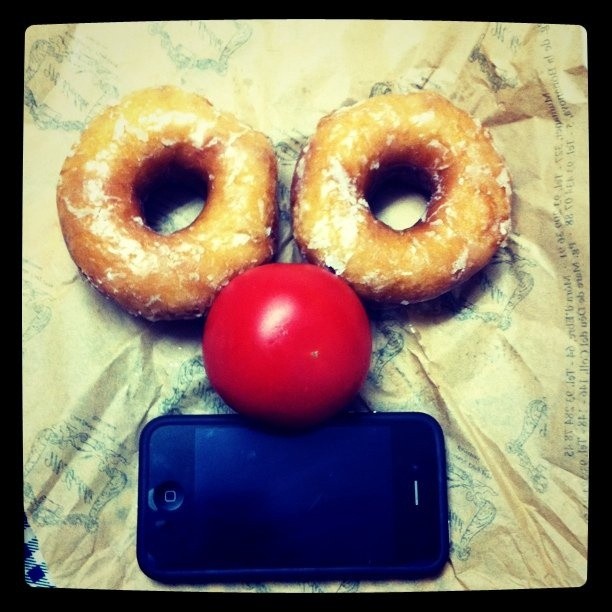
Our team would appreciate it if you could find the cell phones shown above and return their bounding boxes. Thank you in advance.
[137,412,449,580]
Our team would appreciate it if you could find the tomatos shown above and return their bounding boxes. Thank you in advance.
[202,262,372,422]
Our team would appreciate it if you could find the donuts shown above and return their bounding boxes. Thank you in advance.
[290,90,513,306]
[57,86,279,325]
